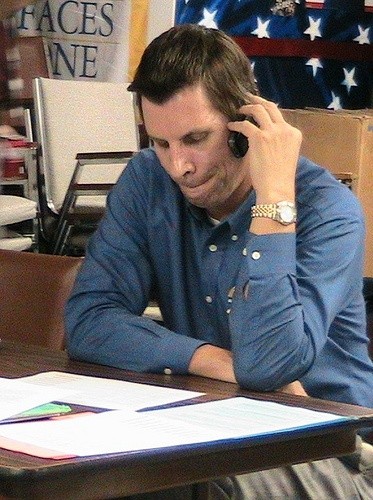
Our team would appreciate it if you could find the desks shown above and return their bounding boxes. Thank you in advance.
[1,336,372,500]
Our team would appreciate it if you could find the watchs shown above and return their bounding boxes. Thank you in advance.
[251,201,297,226]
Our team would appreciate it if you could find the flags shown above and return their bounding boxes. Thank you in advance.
[174,0,372,110]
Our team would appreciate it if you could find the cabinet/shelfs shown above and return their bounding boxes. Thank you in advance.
[0,134,47,264]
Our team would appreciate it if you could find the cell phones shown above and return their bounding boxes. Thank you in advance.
[227,114,259,158]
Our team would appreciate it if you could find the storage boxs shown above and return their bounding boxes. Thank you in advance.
[261,106,372,279]
[0,28,50,103]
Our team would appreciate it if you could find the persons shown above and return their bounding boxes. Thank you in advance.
[62,24,373,500]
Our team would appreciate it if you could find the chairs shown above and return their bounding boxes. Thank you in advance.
[0,247,91,355]
[20,77,142,258]
